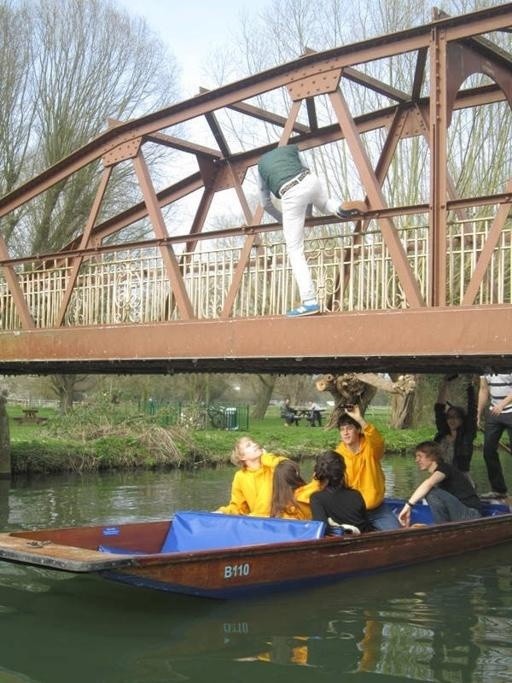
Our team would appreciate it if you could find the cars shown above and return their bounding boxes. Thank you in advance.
[268,398,278,406]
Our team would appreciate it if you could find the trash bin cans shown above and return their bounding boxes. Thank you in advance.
[224,407,237,429]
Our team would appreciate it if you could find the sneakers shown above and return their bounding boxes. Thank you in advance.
[337,201,368,216]
[287,304,321,317]
[481,490,509,499]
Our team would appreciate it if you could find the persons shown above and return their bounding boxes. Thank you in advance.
[396,442,485,526]
[280,398,295,426]
[335,402,385,515]
[310,449,373,536]
[477,373,511,499]
[305,400,321,419]
[209,433,290,519]
[266,460,321,522]
[432,371,477,489]
[257,142,369,319]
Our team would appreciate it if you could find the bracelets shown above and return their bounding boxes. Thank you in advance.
[405,501,415,509]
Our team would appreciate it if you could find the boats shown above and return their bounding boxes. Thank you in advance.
[1,496,511,604]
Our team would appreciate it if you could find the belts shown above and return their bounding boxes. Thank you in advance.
[280,171,310,194]
